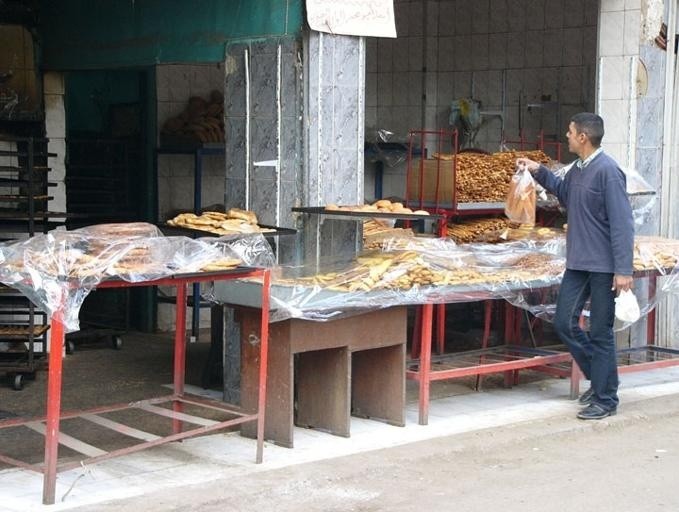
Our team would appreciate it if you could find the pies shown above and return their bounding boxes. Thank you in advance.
[12,208,261,274]
[162,88,224,143]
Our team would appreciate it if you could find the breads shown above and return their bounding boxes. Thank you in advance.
[327,198,430,218]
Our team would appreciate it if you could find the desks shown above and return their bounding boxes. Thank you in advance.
[217,202,678,451]
[1,260,270,507]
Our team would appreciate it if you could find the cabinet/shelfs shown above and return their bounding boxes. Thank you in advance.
[1,134,90,391]
[150,140,424,341]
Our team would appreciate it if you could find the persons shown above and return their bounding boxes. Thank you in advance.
[516,112,635,419]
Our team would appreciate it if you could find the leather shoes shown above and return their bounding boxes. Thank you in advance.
[580,385,595,405]
[577,402,616,419]
